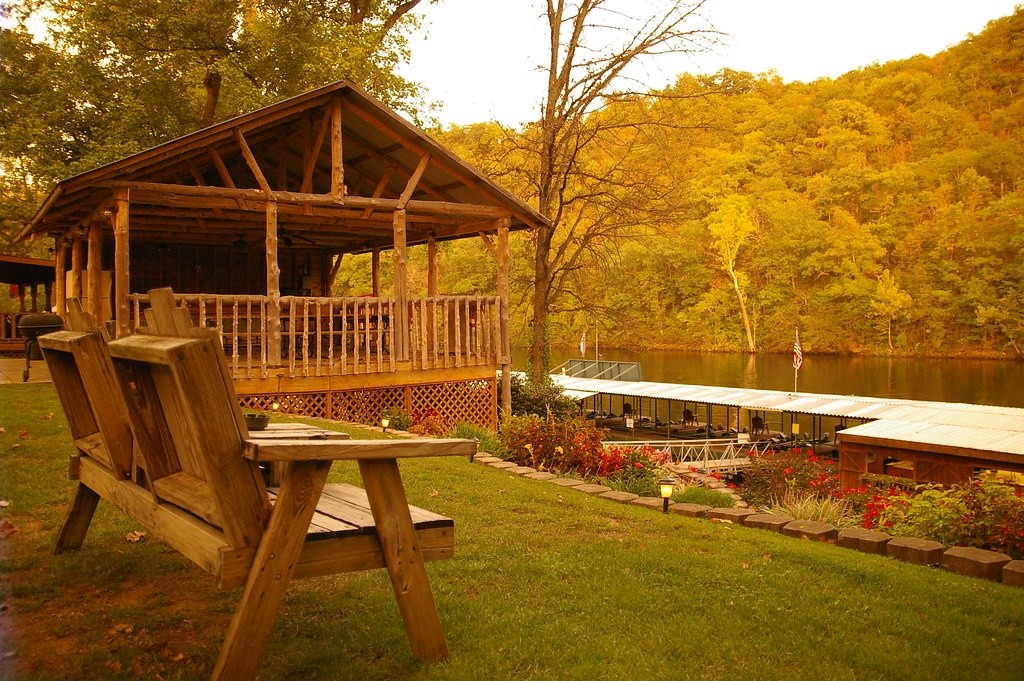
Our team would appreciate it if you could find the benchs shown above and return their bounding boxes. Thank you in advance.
[35,285,476,681]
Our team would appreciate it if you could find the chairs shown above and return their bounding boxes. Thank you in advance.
[751,417,770,435]
[682,409,699,427]
[834,424,848,444]
[622,403,638,420]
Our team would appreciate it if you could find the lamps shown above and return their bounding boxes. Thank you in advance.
[381,414,390,431]
[658,475,676,512]
[272,401,280,412]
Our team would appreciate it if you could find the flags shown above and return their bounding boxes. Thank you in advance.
[580,335,585,357]
[793,331,803,369]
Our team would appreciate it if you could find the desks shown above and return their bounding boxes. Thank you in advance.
[280,317,331,359]
[338,315,391,354]
[246,423,350,440]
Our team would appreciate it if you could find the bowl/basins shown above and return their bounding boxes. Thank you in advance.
[244,413,269,430]
